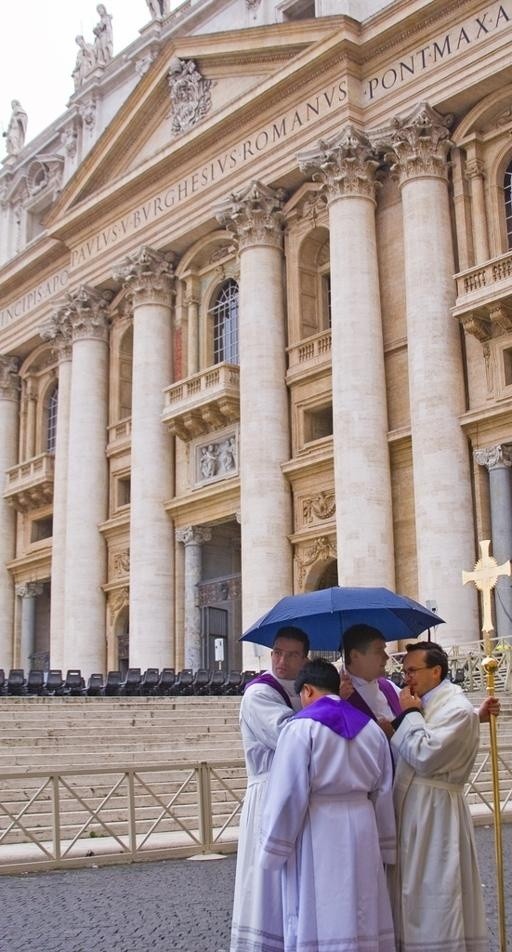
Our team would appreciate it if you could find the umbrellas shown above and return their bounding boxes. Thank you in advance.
[240,584,447,674]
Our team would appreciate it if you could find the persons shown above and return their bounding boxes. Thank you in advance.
[146,0,161,19]
[229,627,310,952]
[93,4,113,66]
[71,35,96,79]
[388,642,489,952]
[2,99,28,156]
[252,656,398,952]
[160,0,170,16]
[337,624,501,924]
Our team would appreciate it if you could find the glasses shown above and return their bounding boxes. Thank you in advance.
[399,666,433,681]
